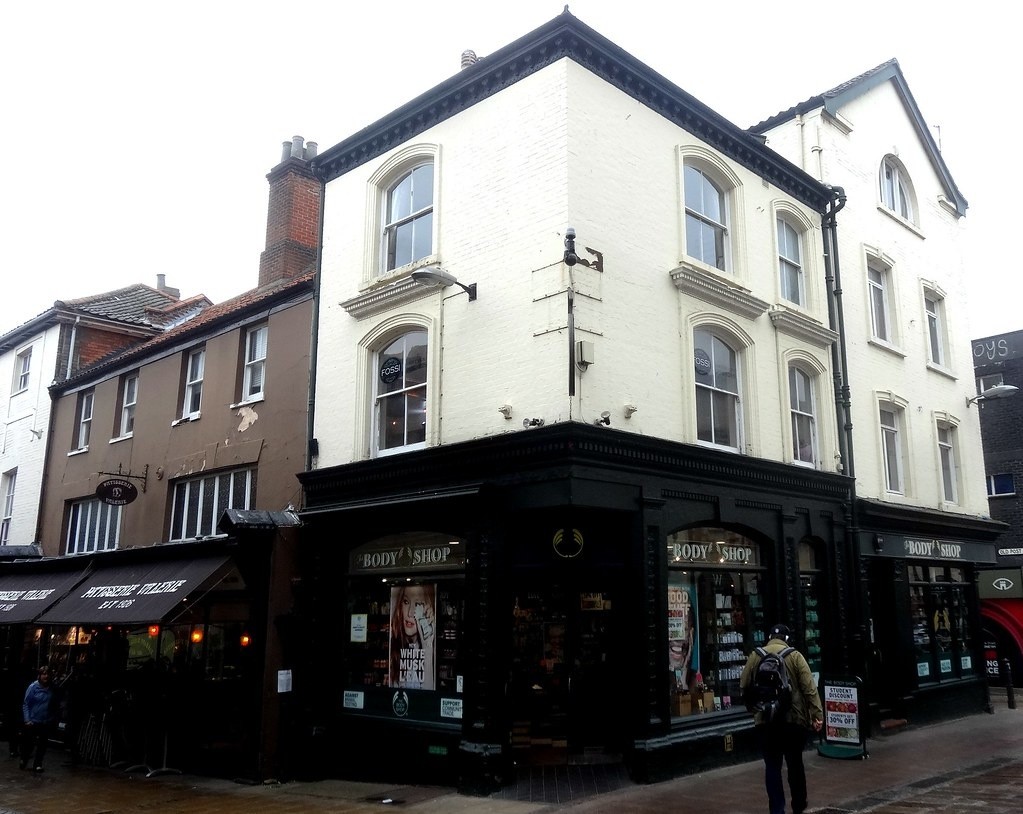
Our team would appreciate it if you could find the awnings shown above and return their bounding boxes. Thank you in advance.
[34,551,242,627]
[0,564,98,627]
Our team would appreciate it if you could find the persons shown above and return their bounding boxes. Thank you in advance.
[669,589,699,689]
[738,624,824,814]
[389,585,436,688]
[17,664,57,773]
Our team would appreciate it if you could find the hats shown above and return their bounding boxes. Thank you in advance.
[771,626,790,636]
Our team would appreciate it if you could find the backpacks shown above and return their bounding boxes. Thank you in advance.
[747,646,796,719]
